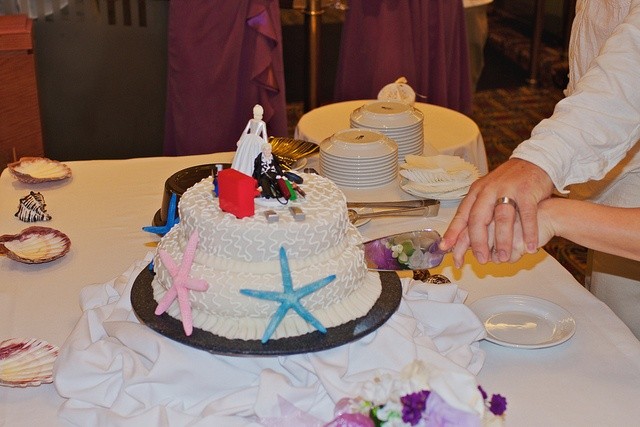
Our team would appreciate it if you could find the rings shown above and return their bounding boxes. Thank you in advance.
[495,197,517,209]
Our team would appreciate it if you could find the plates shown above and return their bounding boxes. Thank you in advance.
[314,128,404,190]
[349,102,426,160]
[460,294,576,351]
[345,203,373,227]
[130,263,403,355]
[289,155,308,171]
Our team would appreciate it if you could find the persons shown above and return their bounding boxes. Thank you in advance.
[252,143,288,199]
[231,104,267,177]
[439,0,640,340]
[453,198,640,270]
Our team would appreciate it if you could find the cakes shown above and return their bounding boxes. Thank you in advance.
[150,104,382,343]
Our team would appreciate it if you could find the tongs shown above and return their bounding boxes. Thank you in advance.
[344,199,442,222]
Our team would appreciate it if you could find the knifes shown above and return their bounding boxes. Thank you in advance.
[354,228,474,272]
